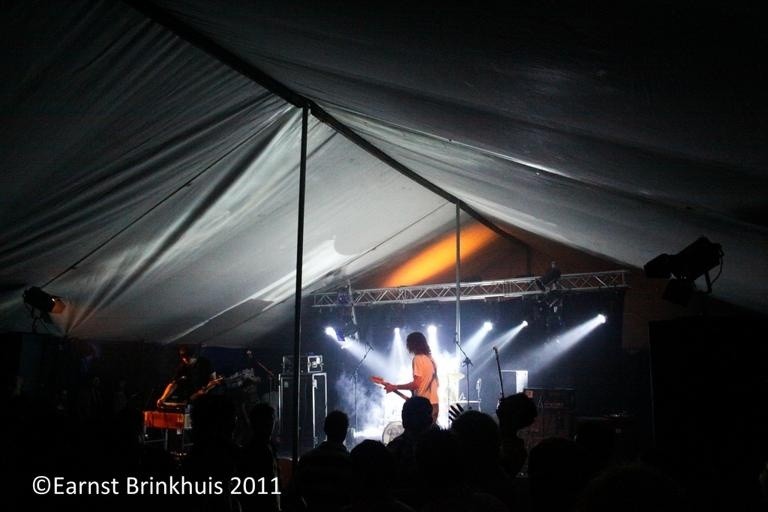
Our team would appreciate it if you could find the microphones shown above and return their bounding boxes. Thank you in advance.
[366,341,375,351]
[454,332,457,344]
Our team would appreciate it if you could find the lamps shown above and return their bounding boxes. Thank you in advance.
[22,285,66,332]
[521,260,566,329]
[644,233,727,305]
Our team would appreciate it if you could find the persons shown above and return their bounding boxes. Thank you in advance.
[155,342,224,411]
[382,331,438,423]
[0,387,768,512]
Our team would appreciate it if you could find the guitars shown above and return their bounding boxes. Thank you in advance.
[372,376,408,401]
